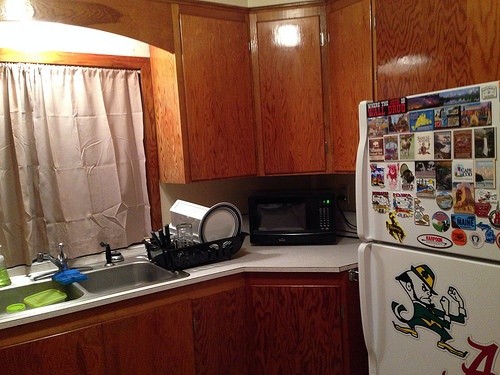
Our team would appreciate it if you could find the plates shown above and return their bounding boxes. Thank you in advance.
[197,202,243,252]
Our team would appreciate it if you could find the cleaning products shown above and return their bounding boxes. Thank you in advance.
[0,244,11,288]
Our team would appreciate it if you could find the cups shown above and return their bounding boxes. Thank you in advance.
[176,222,193,248]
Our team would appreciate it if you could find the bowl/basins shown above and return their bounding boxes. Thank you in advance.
[23,290,67,309]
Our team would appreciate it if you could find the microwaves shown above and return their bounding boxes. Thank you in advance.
[249,189,337,245]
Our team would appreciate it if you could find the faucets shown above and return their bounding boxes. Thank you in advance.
[36,242,69,275]
[100,242,112,267]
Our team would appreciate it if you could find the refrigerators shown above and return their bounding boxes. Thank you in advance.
[355,81,500,375]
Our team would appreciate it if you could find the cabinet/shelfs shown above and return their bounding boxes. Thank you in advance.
[130,0,500,186]
[0,271,360,375]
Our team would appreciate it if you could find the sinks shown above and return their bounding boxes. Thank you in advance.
[0,277,87,330]
[73,255,190,296]
